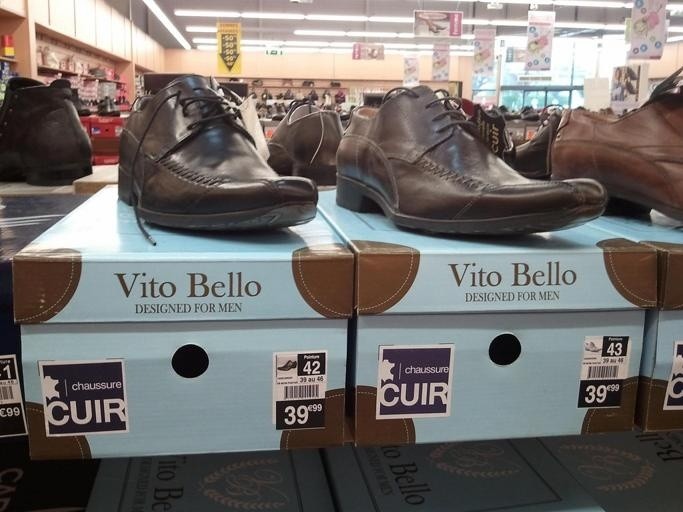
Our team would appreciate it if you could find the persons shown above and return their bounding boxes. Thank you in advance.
[611,68,627,101]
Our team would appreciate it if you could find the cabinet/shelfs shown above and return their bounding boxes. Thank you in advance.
[165,47,470,136]
[129,23,164,113]
[27,0,135,115]
[0,0,37,95]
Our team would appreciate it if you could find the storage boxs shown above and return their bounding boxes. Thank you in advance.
[587,215,683,436]
[320,186,657,448]
[12,181,354,463]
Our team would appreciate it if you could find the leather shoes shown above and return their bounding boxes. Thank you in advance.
[547,72,683,222]
[267,100,342,185]
[0,77,94,185]
[116,73,319,237]
[335,87,607,238]
[510,107,565,177]
